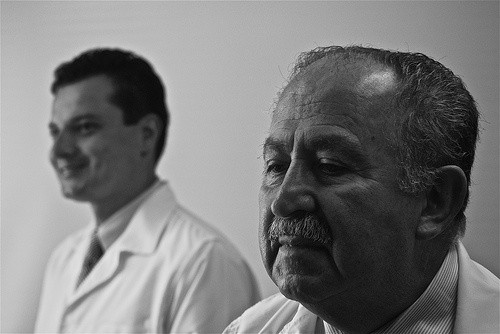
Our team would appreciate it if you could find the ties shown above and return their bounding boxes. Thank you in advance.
[75,229,105,289]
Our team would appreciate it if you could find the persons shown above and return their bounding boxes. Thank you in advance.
[28,45,264,334]
[219,43,500,334]
